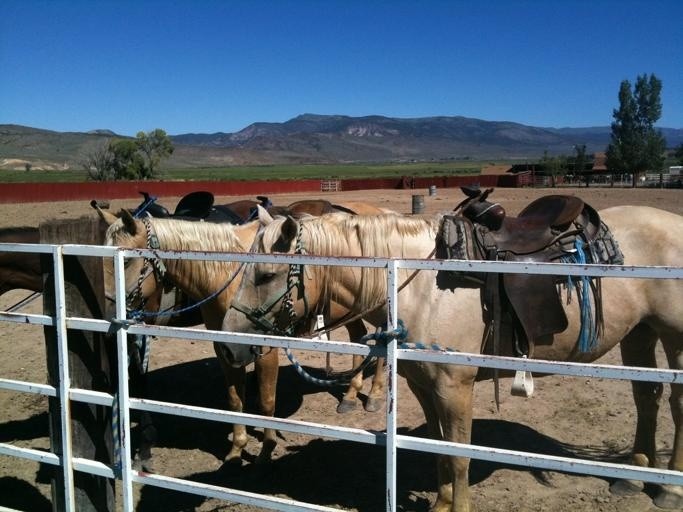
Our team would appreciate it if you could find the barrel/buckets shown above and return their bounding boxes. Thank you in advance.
[412,194,425,215]
[429,185,437,196]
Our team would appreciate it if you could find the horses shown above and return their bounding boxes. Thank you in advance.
[0,201,360,477]
[218,204,683,512]
[90,201,400,482]
